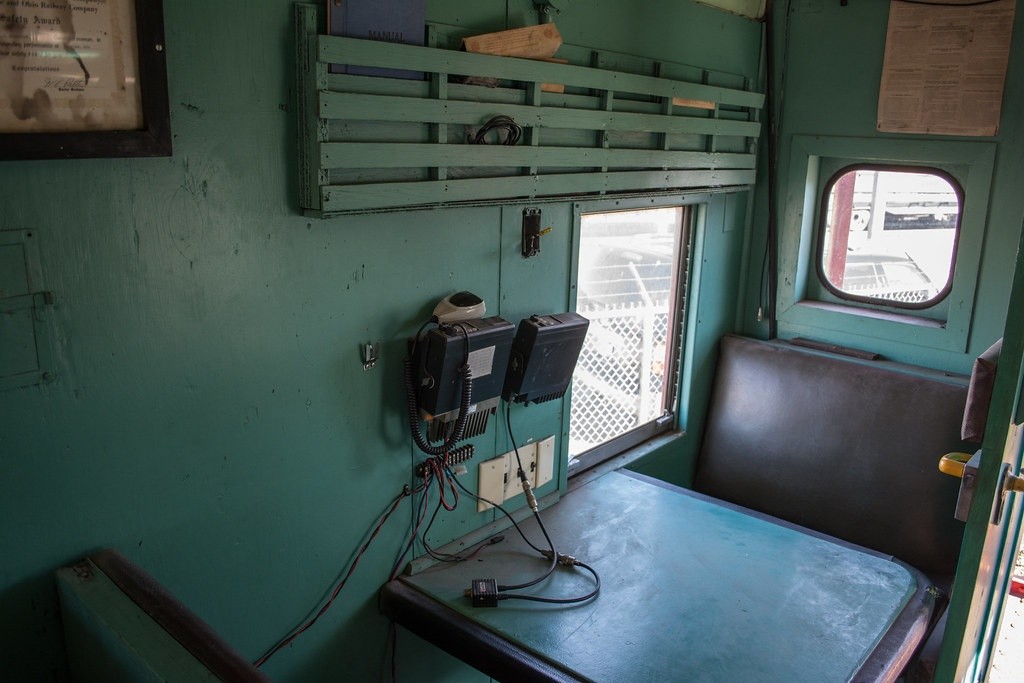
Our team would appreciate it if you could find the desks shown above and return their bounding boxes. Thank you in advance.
[365,468,938,683]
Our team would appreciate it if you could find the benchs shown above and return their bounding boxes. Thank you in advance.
[694,332,977,603]
[0,550,274,683]
